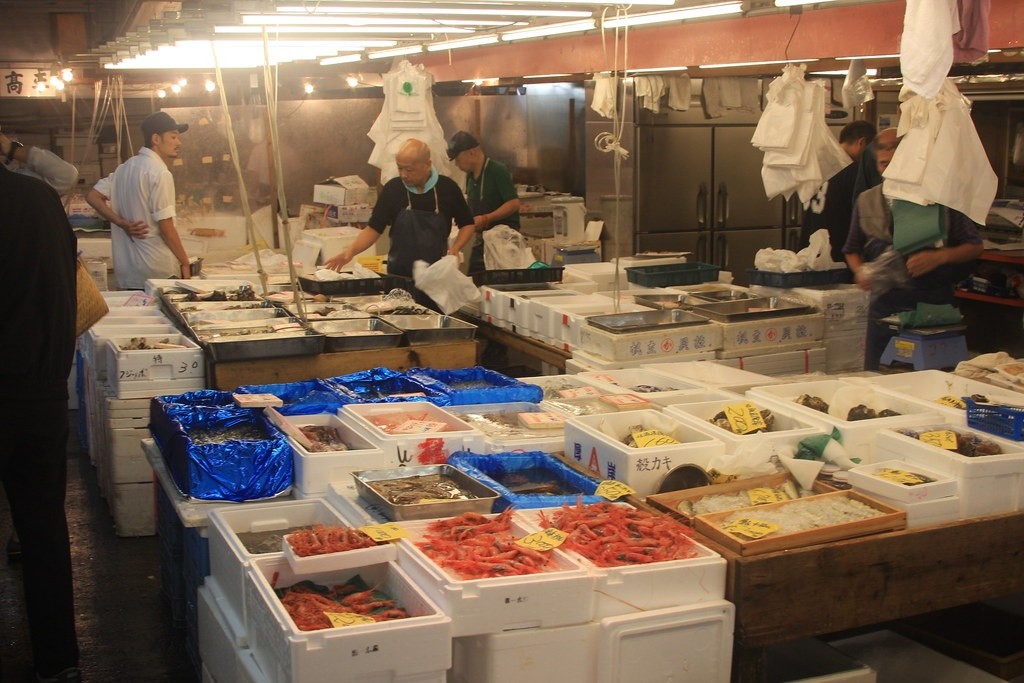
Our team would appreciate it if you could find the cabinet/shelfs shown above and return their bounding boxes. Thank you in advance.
[584,77,865,287]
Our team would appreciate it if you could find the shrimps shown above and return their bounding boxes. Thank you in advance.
[538,490,693,566]
[281,581,405,631]
[370,479,473,503]
[415,506,552,578]
[287,522,376,557]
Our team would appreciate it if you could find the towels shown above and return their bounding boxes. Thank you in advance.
[702,75,760,119]
[952,350,1015,380]
[669,73,691,111]
[590,72,617,119]
[635,74,667,114]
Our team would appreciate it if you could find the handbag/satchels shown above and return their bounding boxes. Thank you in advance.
[76,259,110,334]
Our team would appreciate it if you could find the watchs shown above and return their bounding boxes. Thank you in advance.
[4,140,24,166]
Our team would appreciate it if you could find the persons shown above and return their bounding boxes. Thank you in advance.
[800,120,882,262]
[1,131,81,683]
[322,138,475,294]
[843,128,984,306]
[88,112,190,291]
[447,130,521,272]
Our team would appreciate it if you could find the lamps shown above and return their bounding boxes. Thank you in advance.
[34,0,835,91]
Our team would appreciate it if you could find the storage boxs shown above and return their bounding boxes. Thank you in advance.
[19,128,1024,683]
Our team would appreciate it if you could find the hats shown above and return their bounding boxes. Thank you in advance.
[140,112,188,140]
[445,130,482,162]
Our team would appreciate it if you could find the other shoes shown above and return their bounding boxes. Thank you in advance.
[8,669,82,683]
[6,535,22,556]
